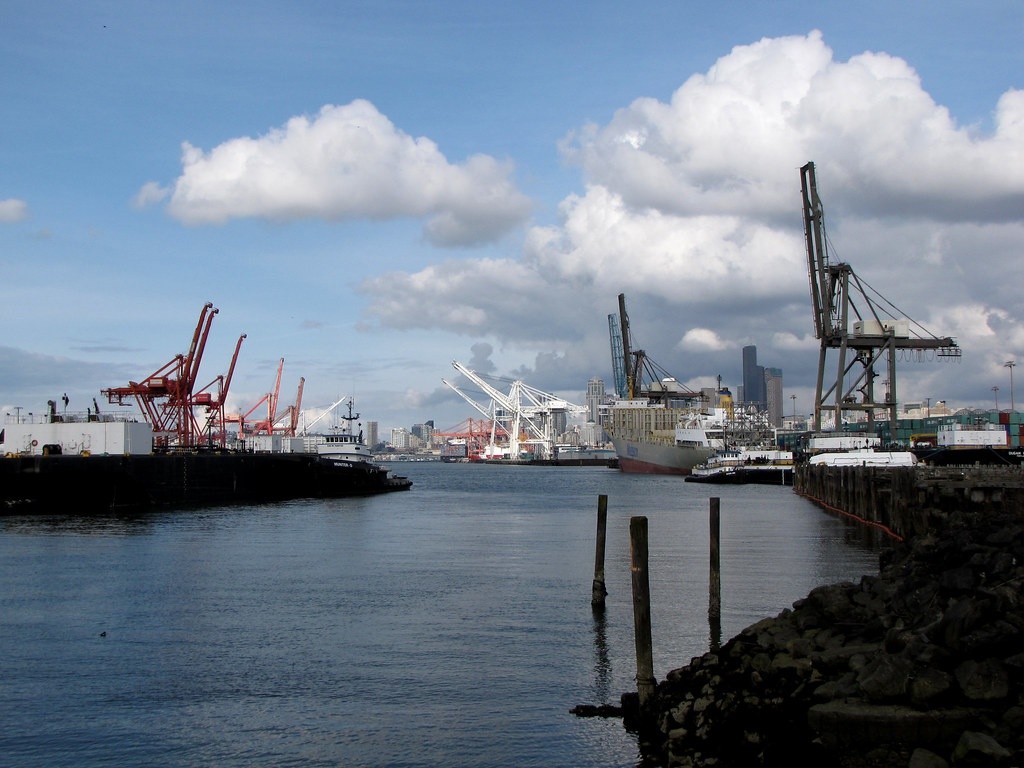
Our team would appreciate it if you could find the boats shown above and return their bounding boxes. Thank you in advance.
[594,397,755,473]
[684,447,741,483]
[317,399,374,464]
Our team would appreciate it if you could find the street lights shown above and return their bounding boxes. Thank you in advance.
[1003,360,1017,411]
[790,394,796,429]
[939,400,946,416]
[14,407,23,423]
[926,397,933,418]
[990,386,1000,412]
[29,413,33,422]
